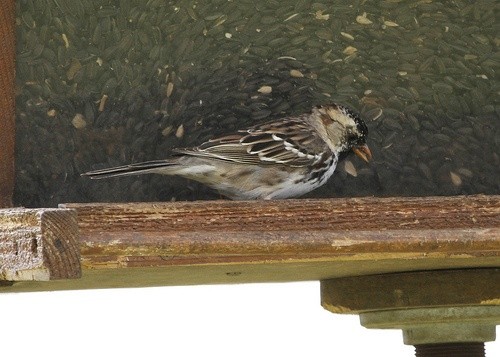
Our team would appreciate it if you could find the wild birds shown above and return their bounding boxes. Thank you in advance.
[79,102,373,201]
